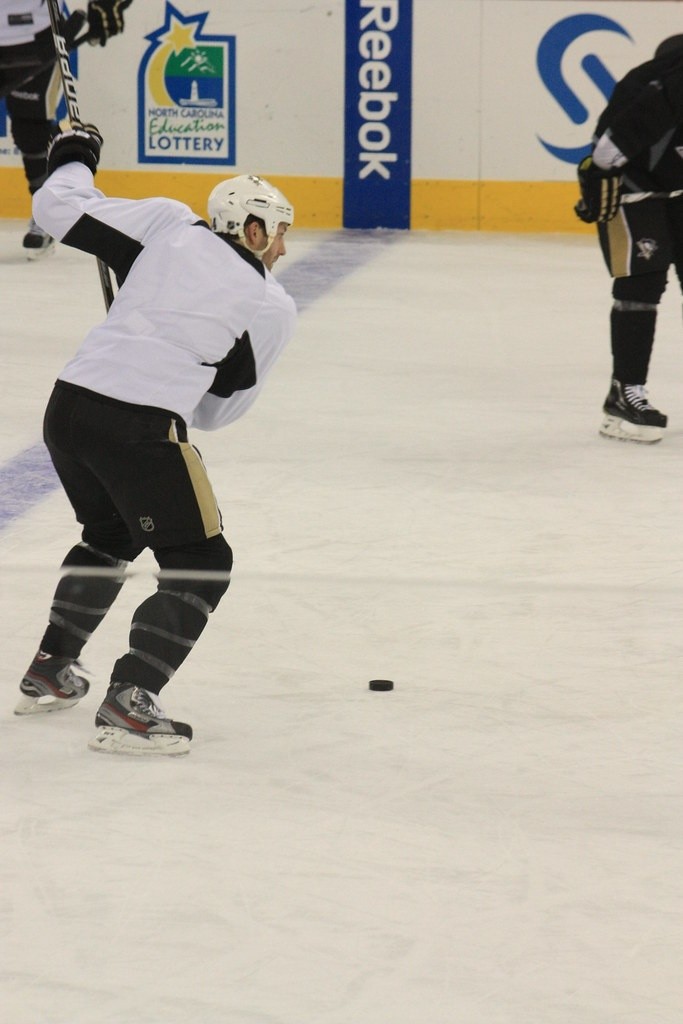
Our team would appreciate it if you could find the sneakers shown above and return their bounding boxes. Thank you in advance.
[599,378,668,442]
[22,219,57,259]
[89,682,193,757]
[14,651,89,715]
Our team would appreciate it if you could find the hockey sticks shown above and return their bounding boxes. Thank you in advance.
[0,31,88,102]
[576,186,683,212]
[45,0,114,311]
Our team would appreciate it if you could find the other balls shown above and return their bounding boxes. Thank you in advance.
[369,679,394,692]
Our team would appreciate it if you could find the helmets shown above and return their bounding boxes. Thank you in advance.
[207,175,295,235]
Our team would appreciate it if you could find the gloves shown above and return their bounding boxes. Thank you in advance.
[574,157,626,222]
[86,0,132,48]
[45,122,102,173]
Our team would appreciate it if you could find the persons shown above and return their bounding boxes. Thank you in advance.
[0,0,133,261]
[573,32,683,445]
[12,117,300,758]
